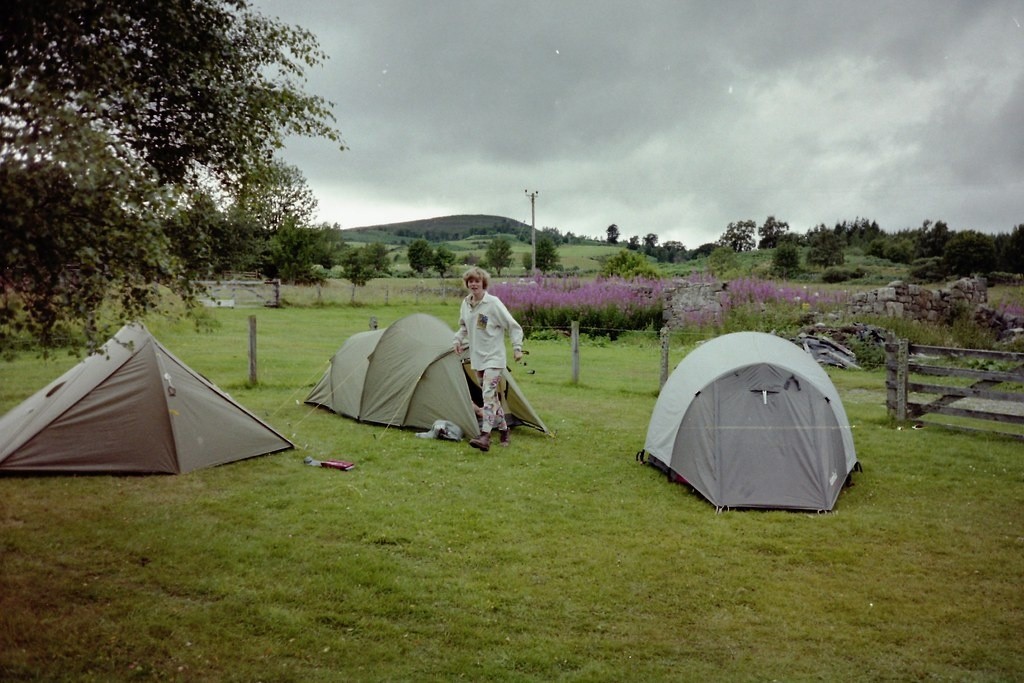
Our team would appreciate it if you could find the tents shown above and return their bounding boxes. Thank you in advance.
[303,311,553,443]
[0,319,293,474]
[642,331,864,515]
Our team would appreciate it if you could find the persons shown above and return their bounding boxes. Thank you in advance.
[450,265,523,451]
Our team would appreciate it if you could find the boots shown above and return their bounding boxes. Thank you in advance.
[469,429,491,451]
[499,427,511,446]
